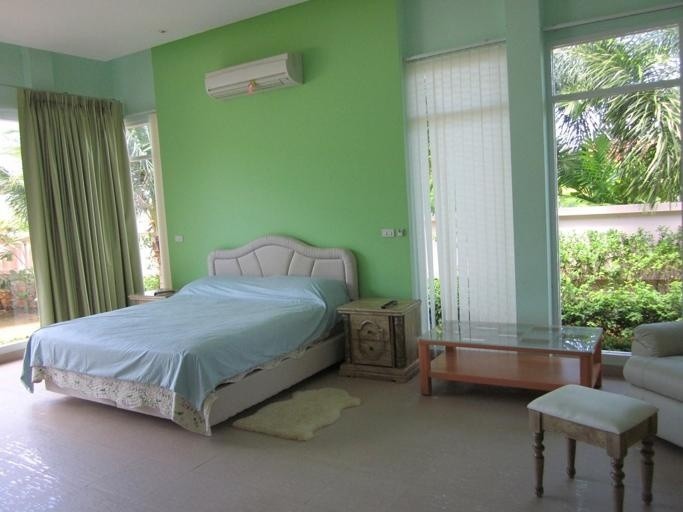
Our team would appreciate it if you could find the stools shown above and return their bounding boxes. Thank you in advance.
[526,383,659,512]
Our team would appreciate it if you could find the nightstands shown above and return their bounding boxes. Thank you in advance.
[128,289,176,306]
[336,296,423,384]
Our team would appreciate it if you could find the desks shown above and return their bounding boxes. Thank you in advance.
[417,319,603,396]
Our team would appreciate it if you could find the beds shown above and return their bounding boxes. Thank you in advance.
[20,233,360,438]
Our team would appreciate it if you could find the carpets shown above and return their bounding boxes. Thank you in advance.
[231,386,362,441]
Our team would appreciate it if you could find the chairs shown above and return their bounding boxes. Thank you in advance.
[622,321,682,449]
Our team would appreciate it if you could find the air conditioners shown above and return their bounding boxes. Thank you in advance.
[203,52,305,101]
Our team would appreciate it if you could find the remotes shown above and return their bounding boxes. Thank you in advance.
[382,300,396,309]
